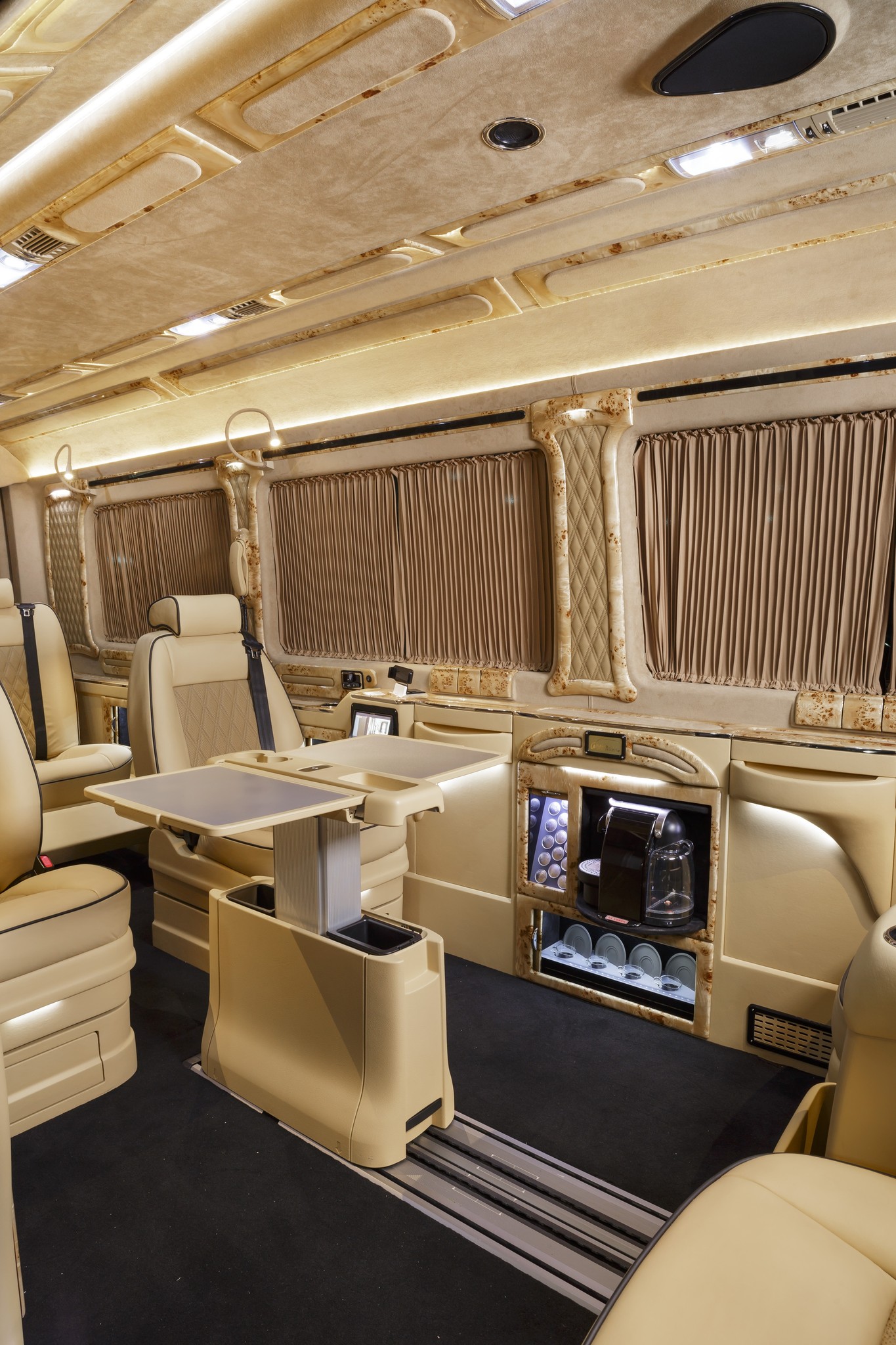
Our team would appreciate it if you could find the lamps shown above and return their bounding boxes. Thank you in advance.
[54,444,97,498]
[225,408,281,472]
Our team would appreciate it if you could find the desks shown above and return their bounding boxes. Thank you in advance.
[80,734,509,1166]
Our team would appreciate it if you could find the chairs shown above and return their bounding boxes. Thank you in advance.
[0,576,133,811]
[0,680,136,1140]
[577,1150,895,1345]
[124,593,423,973]
